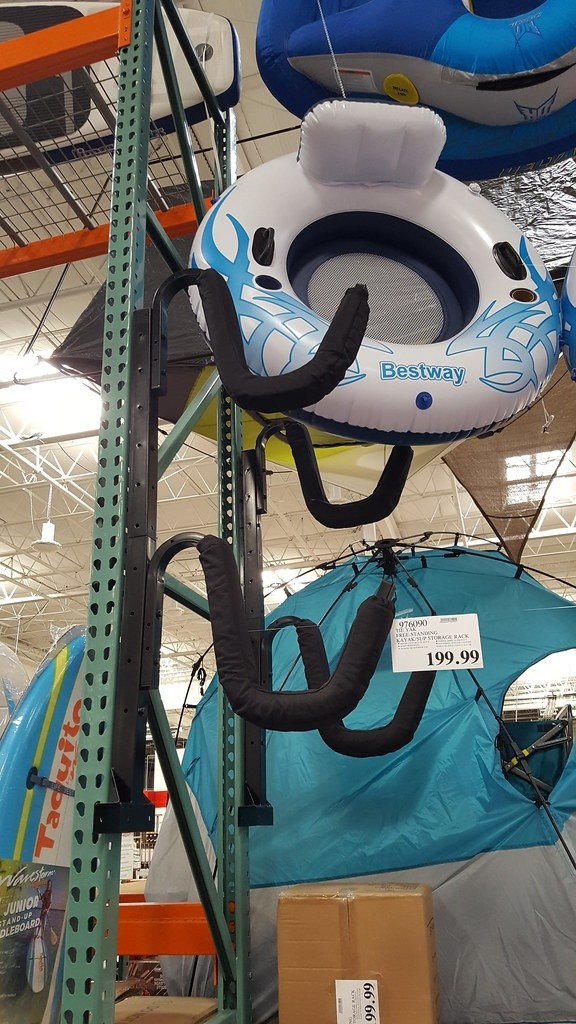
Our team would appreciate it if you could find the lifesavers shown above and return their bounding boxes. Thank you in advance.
[256,0,576,183]
[180,96,565,450]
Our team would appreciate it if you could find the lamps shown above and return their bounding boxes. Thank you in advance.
[29,481,62,554]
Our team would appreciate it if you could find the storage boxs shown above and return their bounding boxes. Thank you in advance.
[276,883,440,1024]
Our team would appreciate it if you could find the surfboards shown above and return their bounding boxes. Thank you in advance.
[0,621,90,1024]
